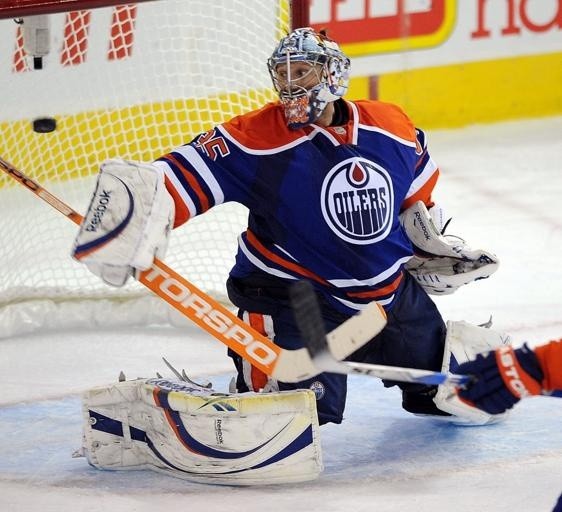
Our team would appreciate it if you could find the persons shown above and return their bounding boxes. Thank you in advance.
[72,25,513,486]
[453,338,562,512]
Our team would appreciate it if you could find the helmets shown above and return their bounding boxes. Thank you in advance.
[271,26,352,102]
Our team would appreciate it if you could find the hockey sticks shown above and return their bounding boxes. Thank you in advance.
[1,160,389,383]
[288,281,562,399]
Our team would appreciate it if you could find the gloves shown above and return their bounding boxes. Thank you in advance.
[433,344,544,426]
